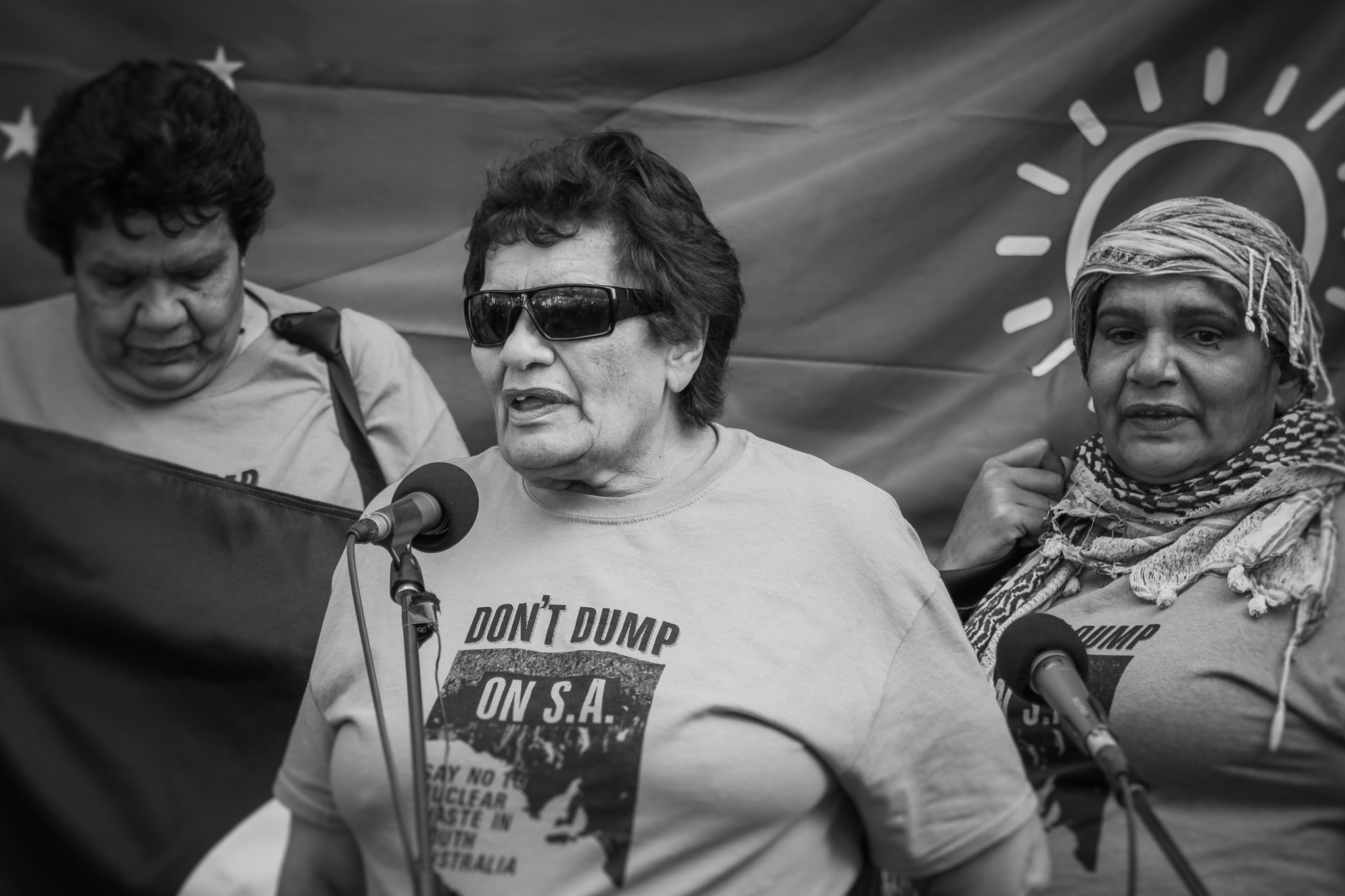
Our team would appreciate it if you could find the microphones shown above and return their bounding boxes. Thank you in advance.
[347,463,480,554]
[995,612,1128,786]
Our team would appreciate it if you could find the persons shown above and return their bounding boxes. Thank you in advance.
[0,56,471,513]
[272,129,1052,896]
[937,195,1345,896]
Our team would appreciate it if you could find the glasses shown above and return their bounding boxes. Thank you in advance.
[461,283,667,348]
